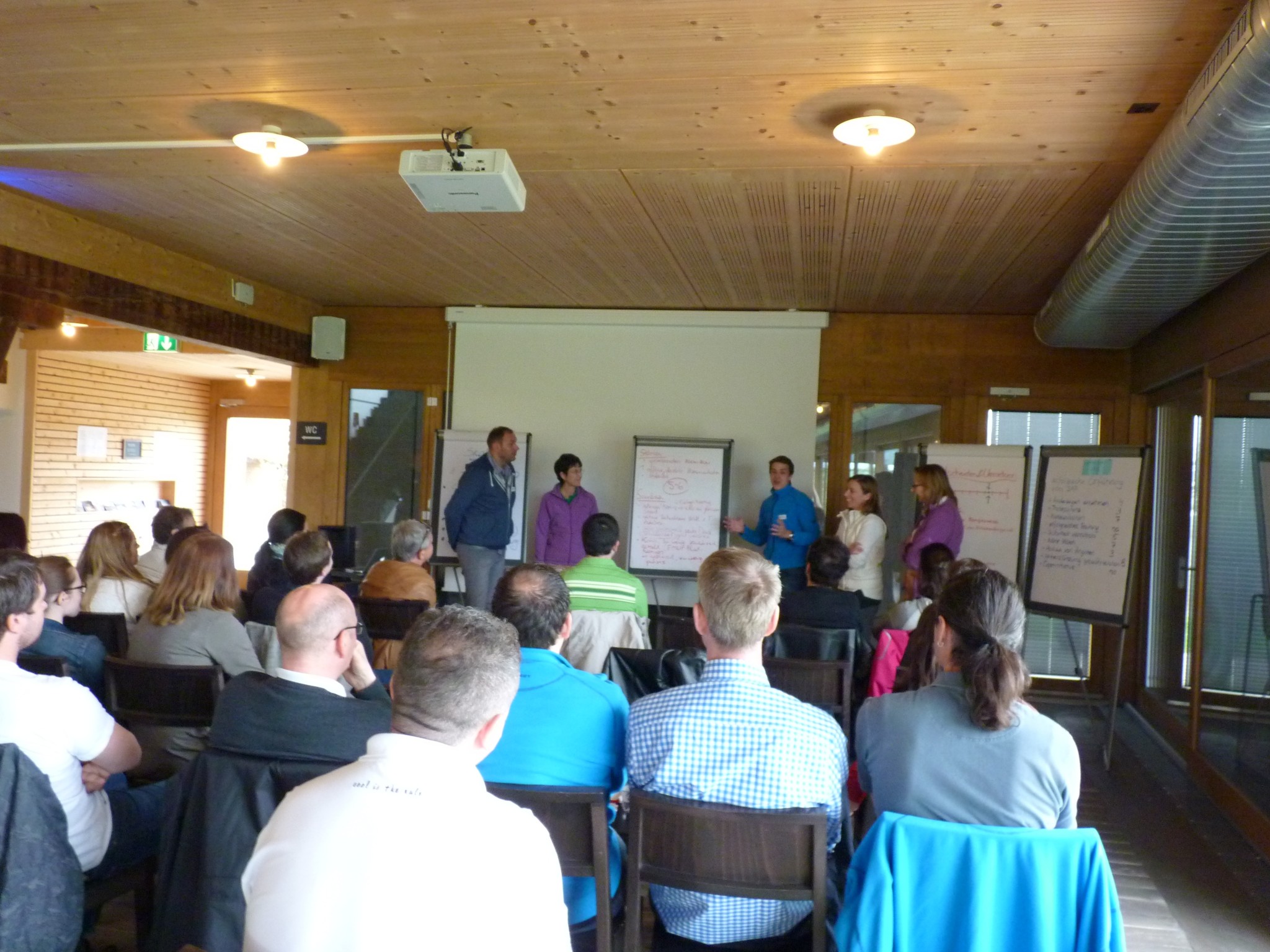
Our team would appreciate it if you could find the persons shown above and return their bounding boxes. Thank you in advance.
[249,531,356,627]
[0,513,31,563]
[77,522,158,620]
[723,455,822,588]
[238,606,579,951]
[835,474,888,609]
[247,508,312,613]
[854,571,1082,829]
[24,554,104,717]
[217,584,399,761]
[900,463,965,601]
[359,520,438,670]
[476,562,630,941]
[623,546,850,952]
[135,507,199,587]
[534,454,599,569]
[128,531,269,774]
[561,511,654,677]
[778,533,875,672]
[164,525,208,565]
[891,556,994,693]
[870,541,956,633]
[444,426,520,610]
[0,550,181,952]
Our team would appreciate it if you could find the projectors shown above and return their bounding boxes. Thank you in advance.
[398,149,527,213]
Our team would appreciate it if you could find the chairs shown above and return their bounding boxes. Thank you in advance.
[20,595,1123,952]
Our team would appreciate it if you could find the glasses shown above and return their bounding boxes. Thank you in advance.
[334,622,362,641]
[912,483,922,488]
[63,583,86,593]
[568,469,584,474]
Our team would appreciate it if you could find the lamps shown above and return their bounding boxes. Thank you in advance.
[60,310,89,339]
[231,125,309,168]
[235,369,267,389]
[832,109,916,157]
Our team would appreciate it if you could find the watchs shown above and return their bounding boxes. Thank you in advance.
[788,530,794,540]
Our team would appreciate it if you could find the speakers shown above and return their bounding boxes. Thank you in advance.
[311,316,346,361]
[318,525,355,568]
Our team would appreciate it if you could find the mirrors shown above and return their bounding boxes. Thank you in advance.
[1131,337,1268,853]
